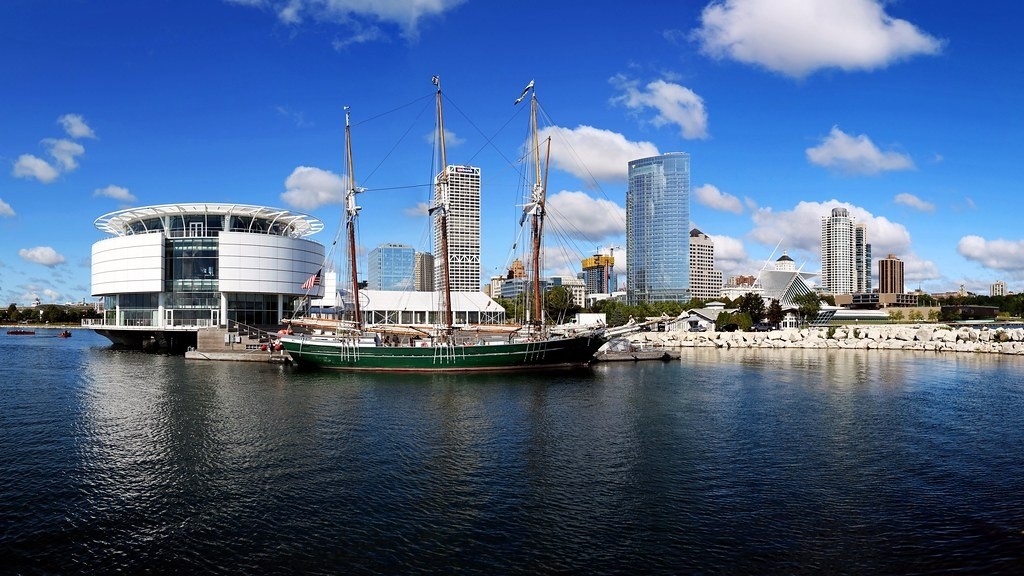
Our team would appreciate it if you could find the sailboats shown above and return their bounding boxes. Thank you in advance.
[277,76,664,375]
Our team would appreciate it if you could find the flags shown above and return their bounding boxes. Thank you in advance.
[301,269,321,290]
[515,79,534,104]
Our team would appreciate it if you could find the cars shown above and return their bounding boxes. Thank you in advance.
[638,325,651,332]
[688,325,707,332]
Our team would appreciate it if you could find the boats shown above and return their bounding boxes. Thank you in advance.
[58,330,72,339]
[6,329,36,335]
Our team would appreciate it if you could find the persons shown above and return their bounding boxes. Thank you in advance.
[392,335,400,347]
[384,335,392,346]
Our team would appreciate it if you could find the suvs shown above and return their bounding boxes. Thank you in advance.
[719,323,738,332]
[748,322,773,332]
